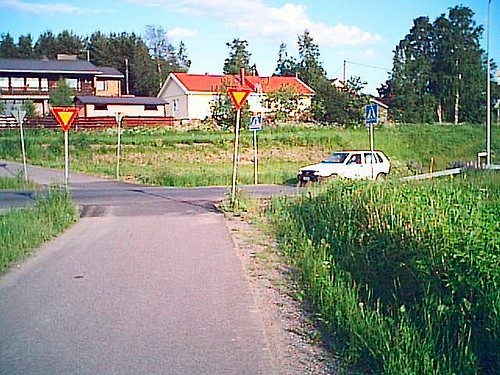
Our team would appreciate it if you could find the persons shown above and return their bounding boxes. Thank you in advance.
[346,156,357,165]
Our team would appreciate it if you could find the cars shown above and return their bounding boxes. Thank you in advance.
[297,149,391,185]
